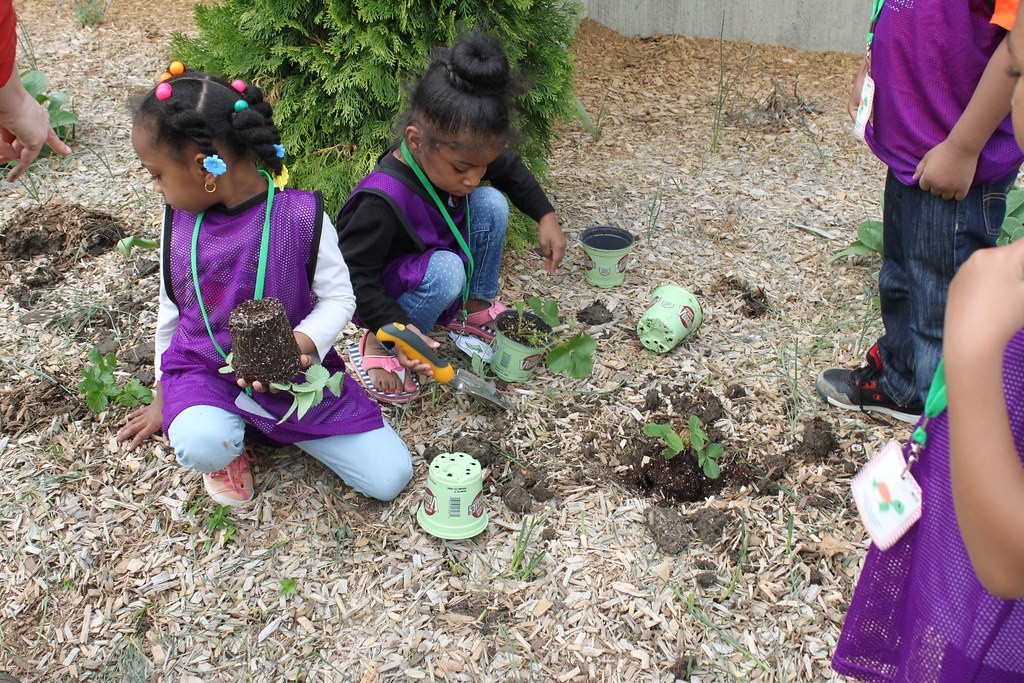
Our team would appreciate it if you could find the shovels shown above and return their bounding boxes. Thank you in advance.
[376,322,512,412]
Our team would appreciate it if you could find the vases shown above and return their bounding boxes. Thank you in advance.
[579,226,634,288]
[416,452,489,540]
[637,282,704,353]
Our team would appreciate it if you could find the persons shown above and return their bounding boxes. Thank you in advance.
[337,32,566,394]
[132,71,413,506]
[815,1,1024,683]
[0,0,71,183]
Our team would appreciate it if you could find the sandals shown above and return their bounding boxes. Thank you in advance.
[348,331,420,404]
[446,300,509,340]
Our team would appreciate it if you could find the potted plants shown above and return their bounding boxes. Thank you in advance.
[469,296,595,383]
[218,299,346,426]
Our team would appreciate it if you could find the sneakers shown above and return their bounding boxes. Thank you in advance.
[815,343,925,425]
[203,447,254,507]
